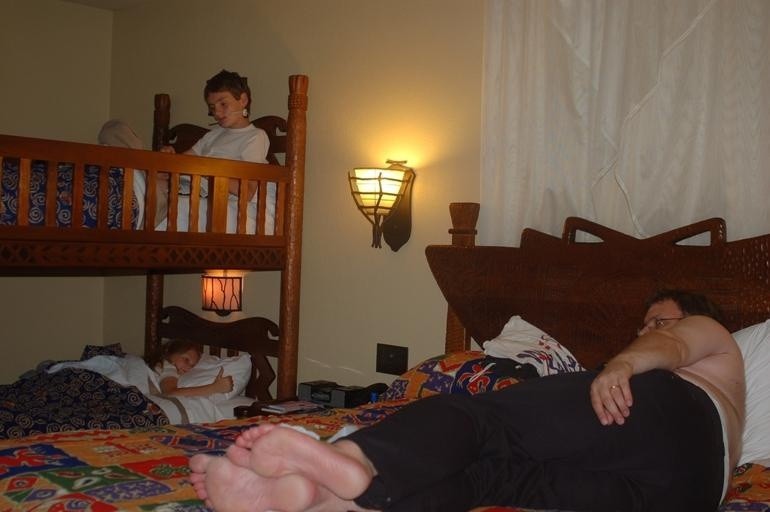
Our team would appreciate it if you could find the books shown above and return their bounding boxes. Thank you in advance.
[261,399,318,414]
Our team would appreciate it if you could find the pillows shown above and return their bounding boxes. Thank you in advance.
[379,351,536,402]
[730,319,770,468]
[177,351,253,401]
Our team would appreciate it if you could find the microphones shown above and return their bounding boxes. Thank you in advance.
[231,109,248,117]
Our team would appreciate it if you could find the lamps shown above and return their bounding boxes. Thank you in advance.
[200,270,245,317]
[348,159,416,253]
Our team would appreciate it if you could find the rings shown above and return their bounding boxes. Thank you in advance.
[609,384,620,391]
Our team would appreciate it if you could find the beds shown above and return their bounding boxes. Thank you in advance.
[0,203,770,512]
[0,75,309,441]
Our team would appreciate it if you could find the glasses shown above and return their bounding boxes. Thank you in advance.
[636,316,683,336]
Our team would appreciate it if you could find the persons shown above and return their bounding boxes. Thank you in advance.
[141,335,235,397]
[97,64,272,236]
[187,282,747,511]
